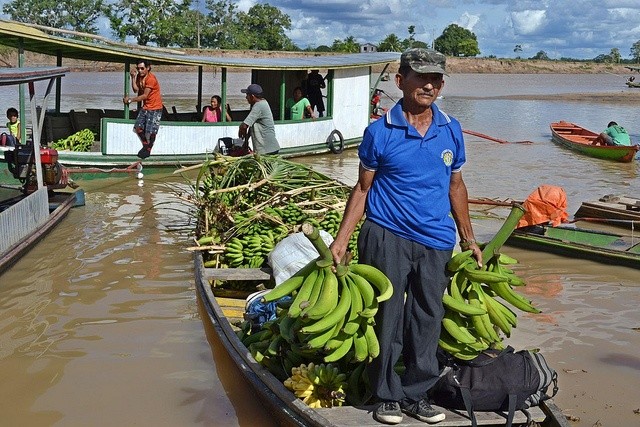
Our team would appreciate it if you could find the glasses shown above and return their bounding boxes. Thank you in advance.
[137,67,145,70]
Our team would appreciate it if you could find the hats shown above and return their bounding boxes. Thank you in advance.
[400,48,449,77]
[241,84,263,96]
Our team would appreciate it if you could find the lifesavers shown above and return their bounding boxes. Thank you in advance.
[330,129,344,153]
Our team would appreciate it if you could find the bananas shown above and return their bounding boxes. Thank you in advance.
[284,363,347,409]
[260,261,393,364]
[195,159,374,290]
[428,250,543,364]
[53,128,96,153]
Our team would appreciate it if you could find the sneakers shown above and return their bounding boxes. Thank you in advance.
[401,399,445,423]
[376,400,403,424]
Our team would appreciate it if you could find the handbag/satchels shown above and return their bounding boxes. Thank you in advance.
[435,345,559,427]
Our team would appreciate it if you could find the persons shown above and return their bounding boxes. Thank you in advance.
[202,96,231,122]
[284,88,316,120]
[306,70,325,118]
[1,108,21,146]
[123,61,163,158]
[324,49,483,424]
[592,121,632,146]
[239,85,280,155]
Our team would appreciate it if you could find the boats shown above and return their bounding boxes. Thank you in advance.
[574,193,640,231]
[192,153,572,427]
[550,120,640,163]
[0,19,403,165]
[0,65,87,275]
[622,65,640,87]
[506,221,640,269]
